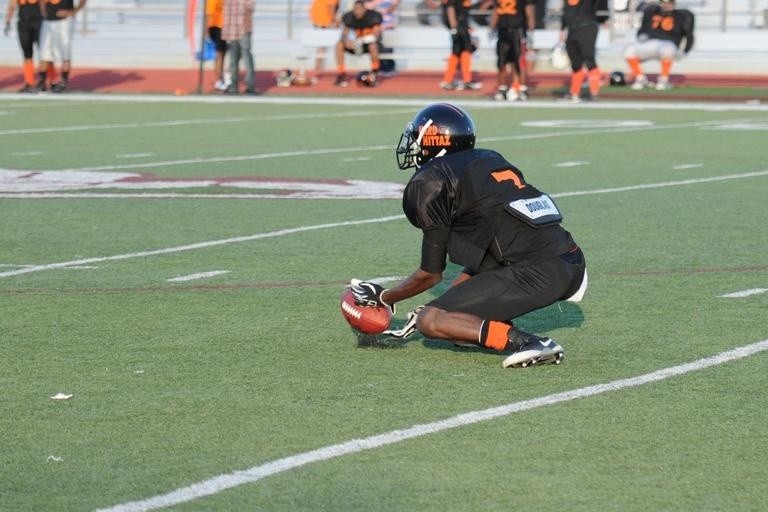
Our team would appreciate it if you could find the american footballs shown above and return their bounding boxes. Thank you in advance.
[340,291,391,334]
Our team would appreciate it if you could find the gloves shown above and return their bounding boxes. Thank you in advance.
[349,279,389,308]
[382,306,428,341]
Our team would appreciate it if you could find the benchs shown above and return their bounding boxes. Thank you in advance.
[292,25,767,80]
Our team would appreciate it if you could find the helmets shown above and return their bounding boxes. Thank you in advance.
[356,70,378,87]
[274,68,292,85]
[398,103,475,172]
[610,72,624,85]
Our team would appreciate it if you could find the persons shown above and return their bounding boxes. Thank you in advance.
[205,1,236,89]
[4,0,62,94]
[311,0,399,86]
[27,0,90,92]
[560,0,608,103]
[625,0,694,91]
[220,0,262,96]
[347,103,588,367]
[418,0,547,102]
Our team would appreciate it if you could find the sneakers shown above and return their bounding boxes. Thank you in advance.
[561,90,598,100]
[503,338,564,368]
[19,70,67,92]
[494,85,528,100]
[438,78,482,90]
[630,75,671,90]
[336,73,345,85]
[215,74,252,93]
